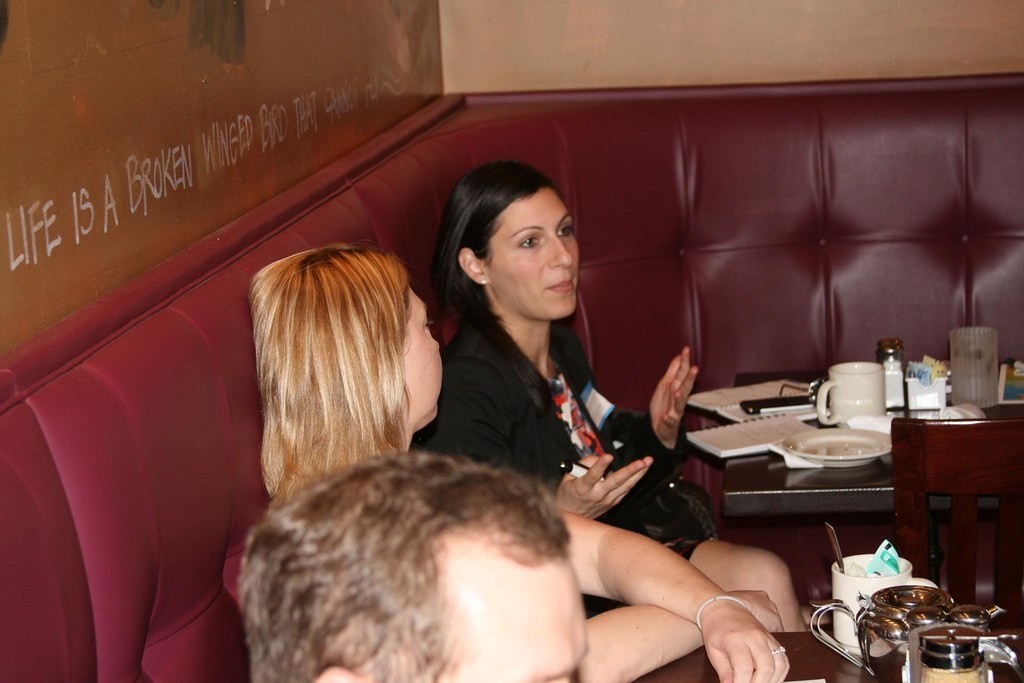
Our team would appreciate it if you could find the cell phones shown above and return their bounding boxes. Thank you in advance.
[739,395,814,415]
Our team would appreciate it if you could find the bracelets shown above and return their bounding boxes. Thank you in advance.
[697,595,747,630]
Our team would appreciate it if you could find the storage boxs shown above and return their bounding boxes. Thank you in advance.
[904,376,949,411]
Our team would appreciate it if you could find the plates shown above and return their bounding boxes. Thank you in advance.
[782,429,892,468]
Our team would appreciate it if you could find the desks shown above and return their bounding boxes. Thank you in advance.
[718,371,1024,588]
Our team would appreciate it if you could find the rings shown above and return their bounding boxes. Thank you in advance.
[772,646,786,656]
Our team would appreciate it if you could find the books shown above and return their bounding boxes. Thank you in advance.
[685,412,820,458]
[687,379,832,423]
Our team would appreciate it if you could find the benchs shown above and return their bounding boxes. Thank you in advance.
[0,74,1024,683]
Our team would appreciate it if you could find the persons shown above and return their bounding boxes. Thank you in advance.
[240,449,588,683]
[415,156,807,632]
[247,242,790,683]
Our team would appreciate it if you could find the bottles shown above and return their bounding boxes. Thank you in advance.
[906,606,949,639]
[875,338,903,365]
[883,349,905,410]
[949,604,991,634]
[916,625,988,683]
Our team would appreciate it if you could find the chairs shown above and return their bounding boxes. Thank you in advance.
[887,418,1024,634]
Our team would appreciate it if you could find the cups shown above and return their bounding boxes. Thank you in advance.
[817,361,885,428]
[830,554,938,656]
[949,326,999,409]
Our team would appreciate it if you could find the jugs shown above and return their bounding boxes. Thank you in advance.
[810,585,1006,683]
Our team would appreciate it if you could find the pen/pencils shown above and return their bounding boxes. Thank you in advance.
[560,460,605,482]
